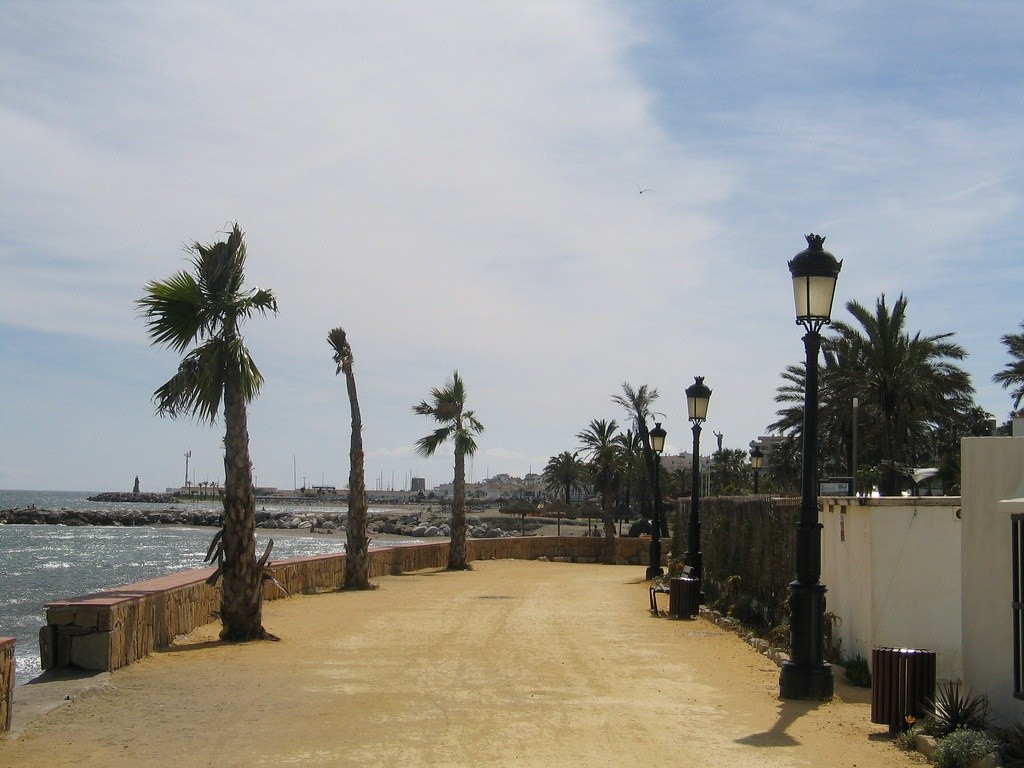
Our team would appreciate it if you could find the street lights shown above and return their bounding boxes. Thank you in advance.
[750,446,764,494]
[645,422,667,580]
[778,232,843,700]
[684,376,712,605]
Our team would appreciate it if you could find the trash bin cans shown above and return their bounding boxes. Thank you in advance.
[870,644,937,725]
[670,578,701,616]
